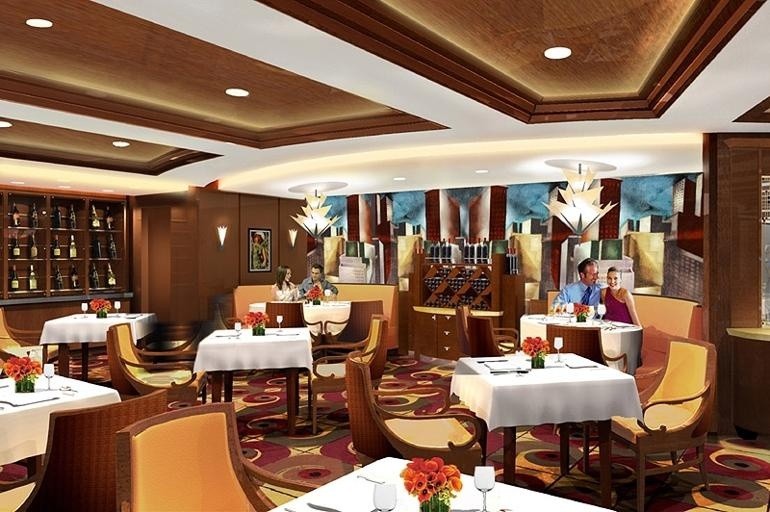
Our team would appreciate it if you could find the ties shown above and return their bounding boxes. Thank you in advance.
[582,288,594,306]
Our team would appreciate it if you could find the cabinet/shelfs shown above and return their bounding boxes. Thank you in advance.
[1,186,128,300]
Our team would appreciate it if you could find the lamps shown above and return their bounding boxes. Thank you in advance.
[216,224,228,247]
[289,228,297,249]
[544,161,618,247]
[293,190,342,246]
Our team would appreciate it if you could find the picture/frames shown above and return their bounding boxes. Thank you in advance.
[248,228,272,274]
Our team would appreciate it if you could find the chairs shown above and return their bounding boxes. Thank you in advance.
[116,402,278,512]
[456,290,643,473]
[308,314,389,435]
[0,299,207,512]
[583,334,718,510]
[234,286,399,376]
[345,350,482,476]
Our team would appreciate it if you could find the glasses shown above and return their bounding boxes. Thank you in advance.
[584,271,599,277]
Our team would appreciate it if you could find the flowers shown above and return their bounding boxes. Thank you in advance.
[400,458,463,509]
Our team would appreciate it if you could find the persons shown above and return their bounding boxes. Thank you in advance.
[296,263,339,299]
[550,257,627,320]
[599,266,642,327]
[269,264,299,302]
